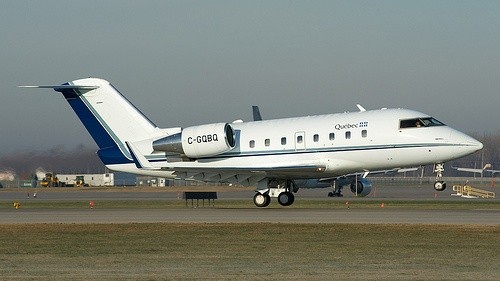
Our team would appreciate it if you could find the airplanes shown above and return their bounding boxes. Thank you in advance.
[16,77,484,207]
